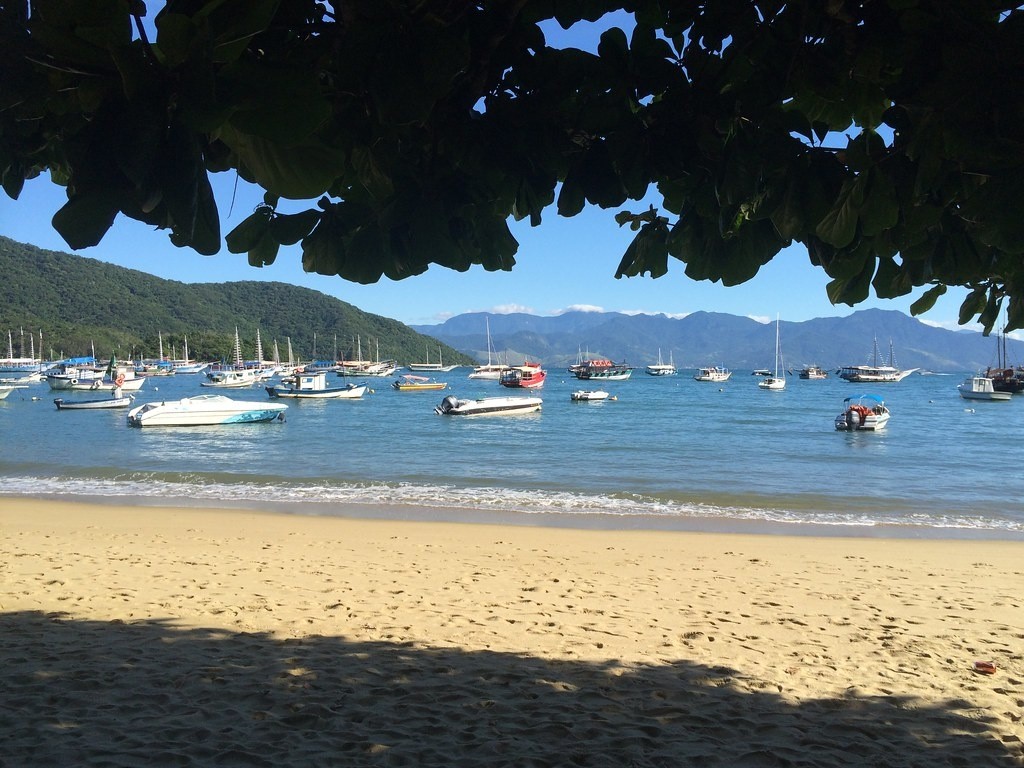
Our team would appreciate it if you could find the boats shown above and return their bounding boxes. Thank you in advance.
[835,394,890,432]
[575,359,633,380]
[433,394,543,418]
[498,361,547,388]
[958,378,1012,401]
[571,388,610,400]
[645,364,676,376]
[52,389,132,410]
[265,363,369,399]
[126,395,288,426]
[0,385,29,399]
[390,375,448,391]
[692,362,733,381]
[202,371,255,388]
[751,370,773,376]
[408,363,443,372]
[44,373,146,390]
[793,362,828,379]
[568,343,584,373]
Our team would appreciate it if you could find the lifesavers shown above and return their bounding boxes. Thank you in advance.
[297,367,305,372]
[115,377,124,386]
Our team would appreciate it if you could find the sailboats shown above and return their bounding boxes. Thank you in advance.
[759,311,786,389]
[835,331,920,383]
[0,325,405,393]
[979,324,1024,394]
[468,316,521,379]
